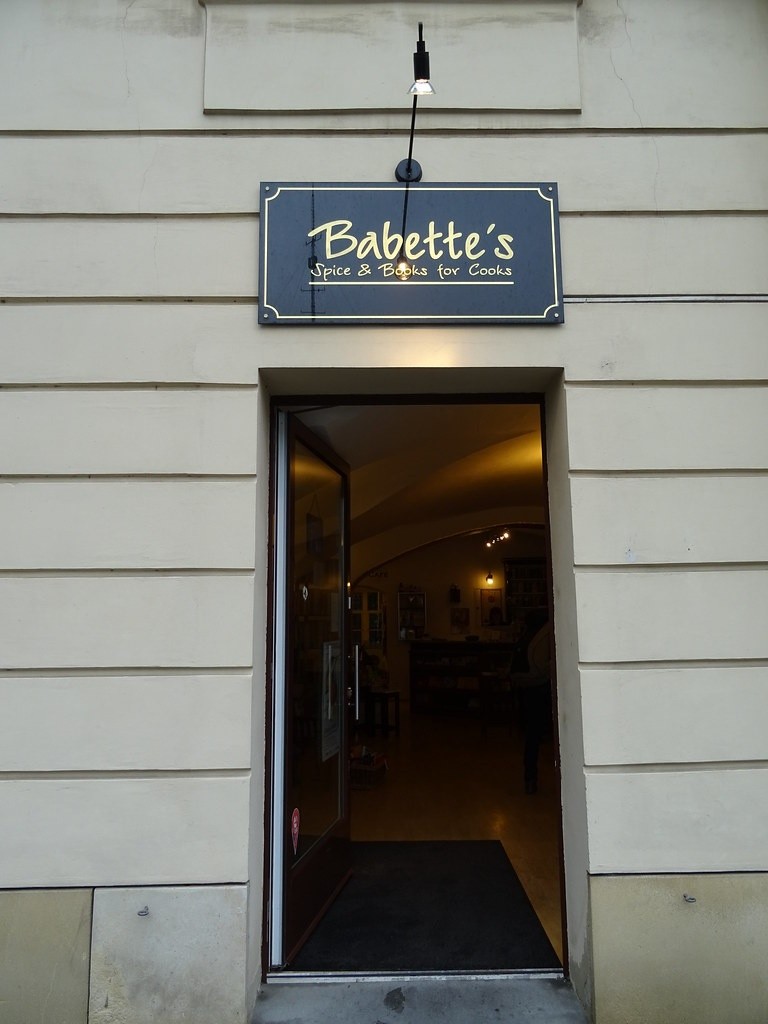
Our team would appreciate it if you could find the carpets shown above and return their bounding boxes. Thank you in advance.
[283,840,563,973]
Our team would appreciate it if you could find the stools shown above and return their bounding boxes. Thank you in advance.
[365,690,400,738]
